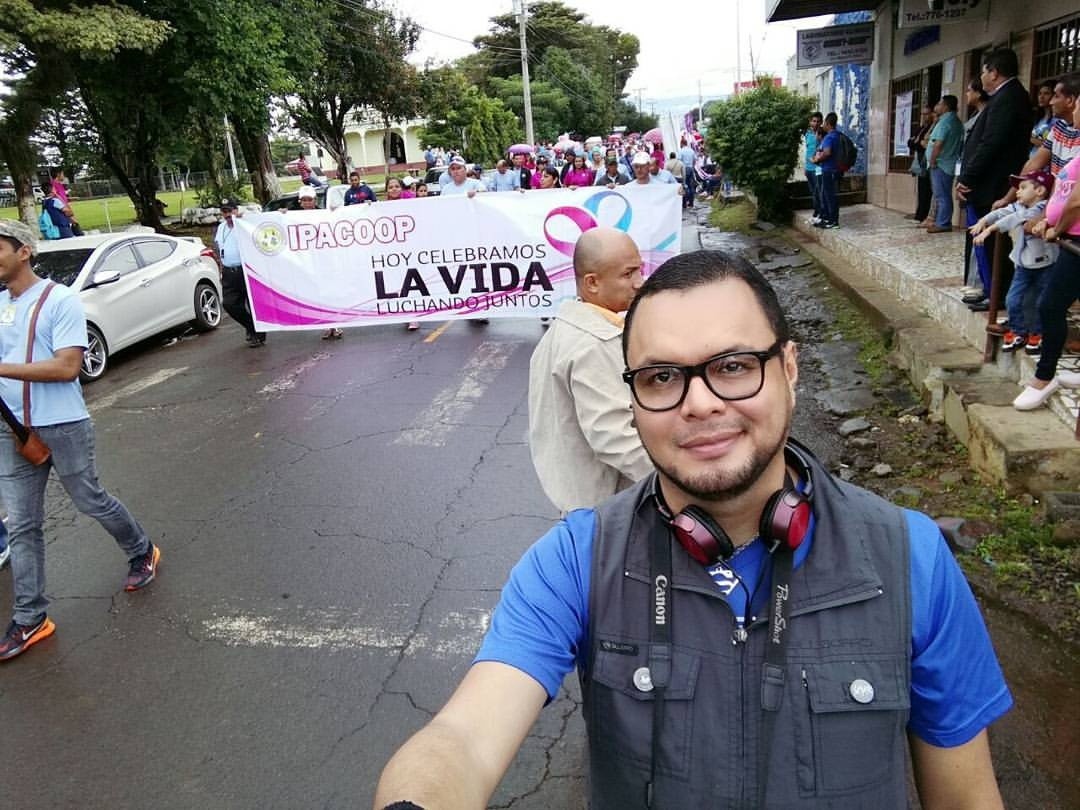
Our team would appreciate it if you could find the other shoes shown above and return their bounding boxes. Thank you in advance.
[984,320,1010,336]
[903,212,926,222]
[908,159,922,174]
[968,299,991,312]
[927,227,952,234]
[408,321,421,330]
[540,317,552,326]
[804,217,822,224]
[0,545,10,567]
[961,295,987,304]
[480,318,489,322]
[916,218,936,228]
[245,334,266,347]
[814,220,840,229]
[322,328,344,340]
[1013,379,1058,411]
[960,286,983,295]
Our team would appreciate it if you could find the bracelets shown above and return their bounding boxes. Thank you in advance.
[383,800,424,810]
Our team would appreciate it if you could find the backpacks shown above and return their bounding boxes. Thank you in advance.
[39,197,61,240]
[829,131,858,176]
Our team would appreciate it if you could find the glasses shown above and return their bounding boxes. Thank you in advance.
[299,197,313,202]
[622,335,790,413]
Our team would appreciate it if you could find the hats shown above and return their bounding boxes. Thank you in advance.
[0,217,38,258]
[632,152,650,165]
[449,156,465,167]
[299,186,316,199]
[219,197,237,209]
[1009,169,1056,192]
[607,157,617,165]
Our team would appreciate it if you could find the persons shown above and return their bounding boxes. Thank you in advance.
[0,516,11,569]
[527,227,656,522]
[0,216,160,665]
[47,166,85,236]
[276,185,343,341]
[383,133,719,332]
[344,171,377,206]
[804,112,858,229]
[370,251,1015,810]
[213,197,267,349]
[39,183,80,240]
[296,152,321,187]
[907,49,1080,412]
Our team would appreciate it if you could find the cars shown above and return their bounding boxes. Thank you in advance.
[0,232,226,384]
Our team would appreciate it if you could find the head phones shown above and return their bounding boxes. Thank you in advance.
[650,445,817,566]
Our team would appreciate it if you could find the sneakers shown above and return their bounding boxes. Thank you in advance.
[123,539,161,591]
[1025,333,1043,355]
[0,611,55,661]
[1002,331,1030,352]
